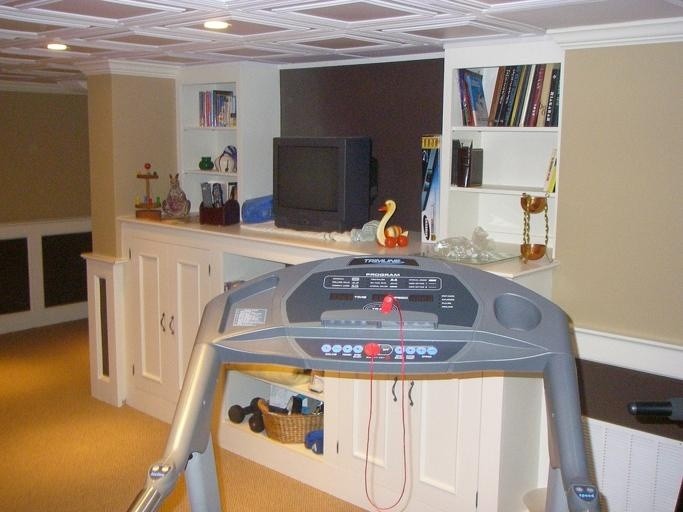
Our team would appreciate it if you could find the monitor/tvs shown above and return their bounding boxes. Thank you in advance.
[273,137,378,233]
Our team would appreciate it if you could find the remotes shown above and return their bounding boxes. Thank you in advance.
[202,182,212,208]
[212,183,223,208]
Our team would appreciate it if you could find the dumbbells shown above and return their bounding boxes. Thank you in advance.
[229,397,264,424]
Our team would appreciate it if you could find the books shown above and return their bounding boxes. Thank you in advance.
[457,62,562,127]
[200,90,234,127]
[454,137,483,186]
[543,141,556,191]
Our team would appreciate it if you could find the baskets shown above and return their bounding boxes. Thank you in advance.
[257,399,324,443]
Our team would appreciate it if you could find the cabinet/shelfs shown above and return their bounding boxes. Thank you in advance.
[337,366,543,512]
[176,59,281,214]
[123,224,210,423]
[441,46,564,251]
